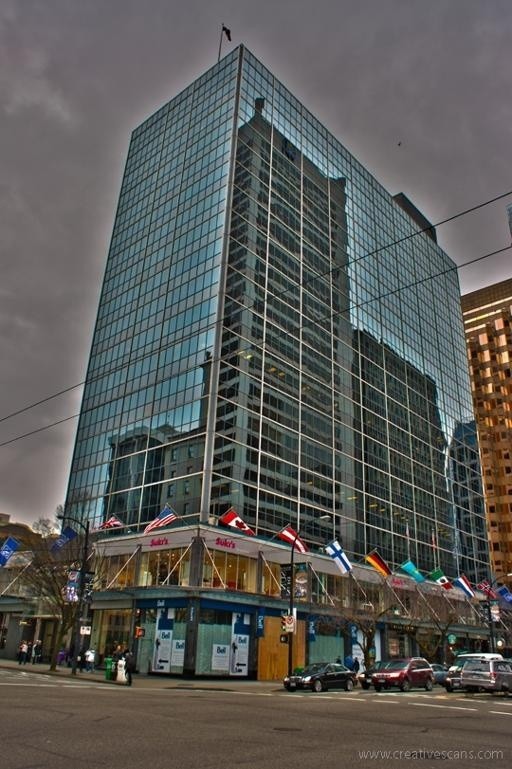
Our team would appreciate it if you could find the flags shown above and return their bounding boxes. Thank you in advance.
[143,504,177,536]
[453,574,475,599]
[364,549,393,577]
[324,538,353,575]
[476,578,497,600]
[399,559,425,583]
[220,509,256,537]
[92,516,122,530]
[48,526,78,554]
[0,535,22,566]
[277,525,309,554]
[496,585,512,602]
[428,567,453,591]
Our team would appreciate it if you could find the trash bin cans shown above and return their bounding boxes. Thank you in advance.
[294,666,304,675]
[103,658,120,681]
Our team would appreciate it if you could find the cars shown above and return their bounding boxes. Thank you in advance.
[284,663,358,691]
[358,657,448,692]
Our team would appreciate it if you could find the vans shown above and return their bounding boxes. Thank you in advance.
[446,653,511,693]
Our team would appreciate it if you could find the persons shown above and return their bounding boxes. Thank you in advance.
[336,655,360,674]
[17,638,135,686]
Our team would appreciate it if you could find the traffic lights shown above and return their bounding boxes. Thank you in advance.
[281,615,287,631]
[136,627,145,637]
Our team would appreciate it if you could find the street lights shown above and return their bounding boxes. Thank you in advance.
[55,514,91,673]
[287,514,331,674]
[487,573,512,652]
